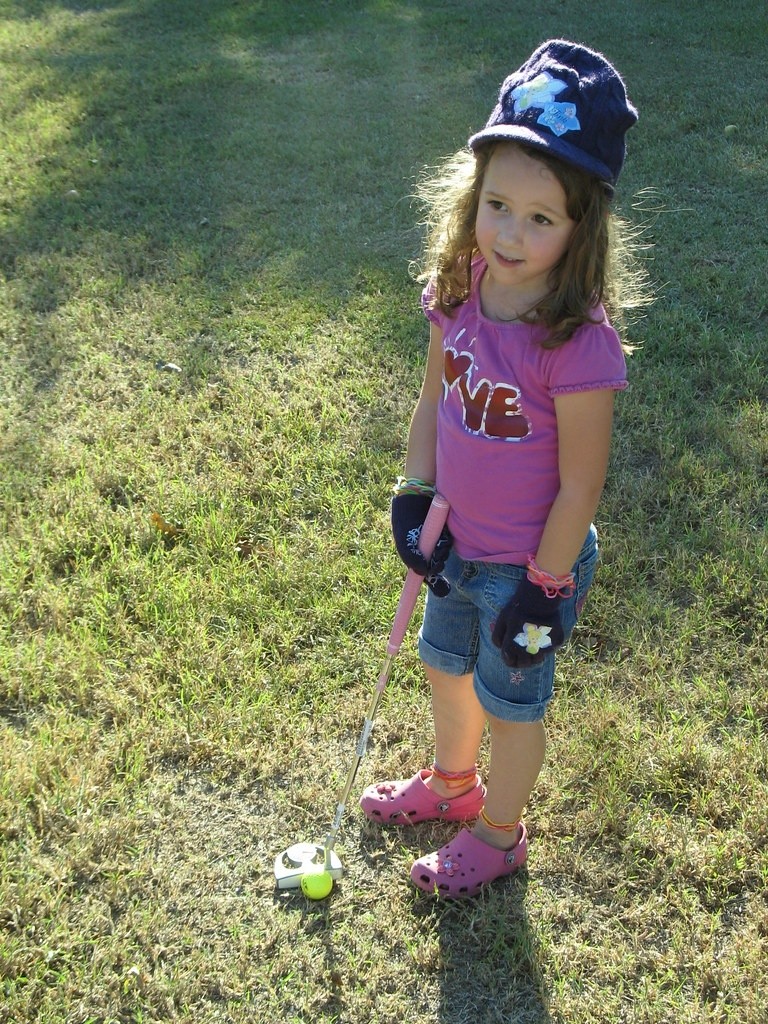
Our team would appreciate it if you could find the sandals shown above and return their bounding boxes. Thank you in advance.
[358,769,488,824]
[410,821,531,900]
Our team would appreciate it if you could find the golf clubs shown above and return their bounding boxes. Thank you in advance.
[272,490,446,890]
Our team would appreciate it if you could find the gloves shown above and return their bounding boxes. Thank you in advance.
[392,495,455,597]
[493,573,564,670]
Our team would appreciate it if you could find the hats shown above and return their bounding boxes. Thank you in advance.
[468,38,638,186]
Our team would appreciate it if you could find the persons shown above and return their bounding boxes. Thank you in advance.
[358,39,638,898]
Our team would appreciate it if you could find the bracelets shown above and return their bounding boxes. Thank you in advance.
[392,475,436,498]
[524,554,577,600]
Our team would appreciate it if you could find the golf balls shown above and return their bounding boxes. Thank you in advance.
[299,868,334,901]
